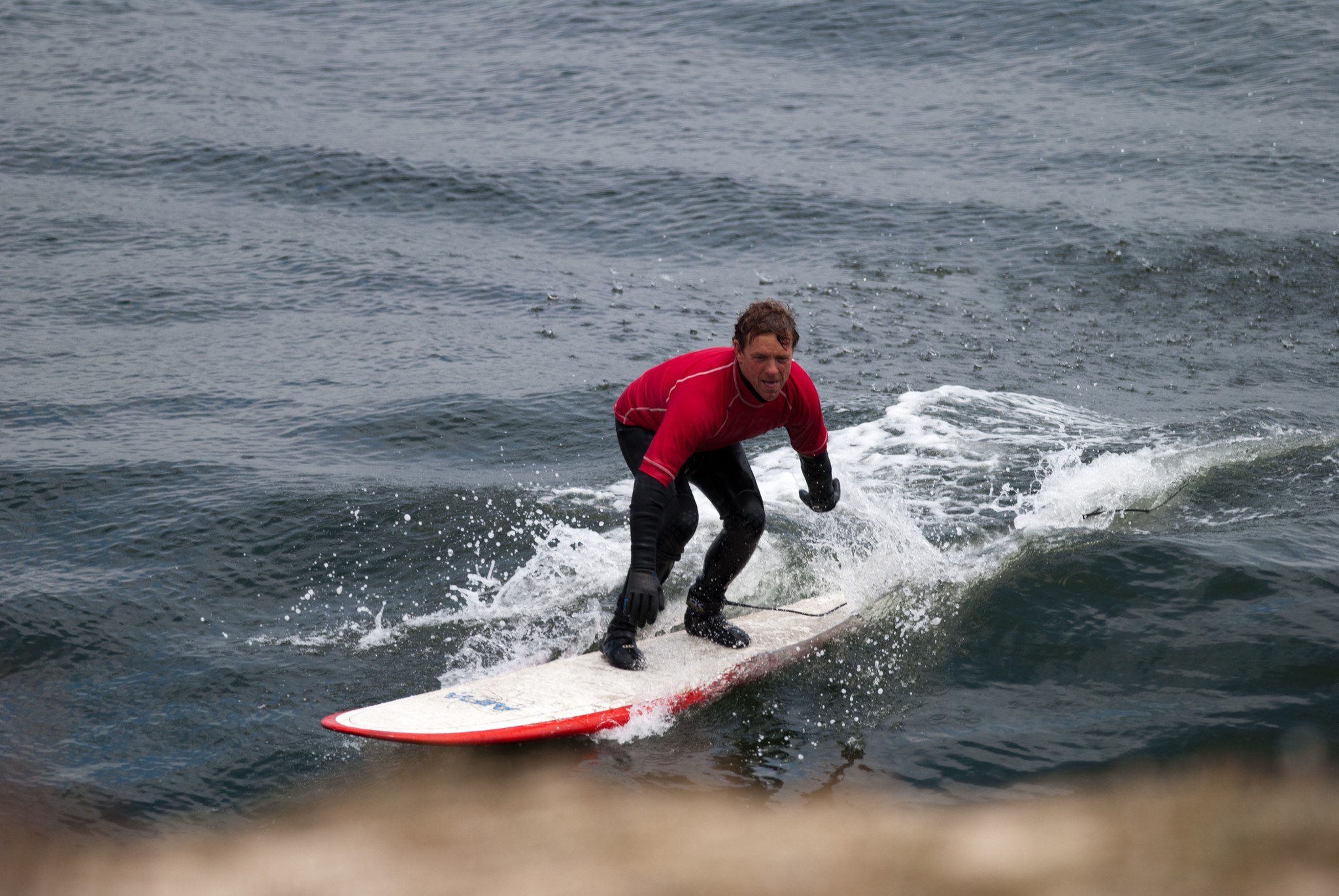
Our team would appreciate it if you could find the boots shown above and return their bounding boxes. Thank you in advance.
[684,578,751,649]
[600,592,648,671]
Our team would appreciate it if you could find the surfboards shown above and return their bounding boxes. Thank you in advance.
[320,591,875,745]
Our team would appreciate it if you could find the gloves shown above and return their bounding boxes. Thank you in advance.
[623,473,665,627]
[799,452,840,512]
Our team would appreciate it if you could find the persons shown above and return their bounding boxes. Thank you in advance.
[601,301,840,670]
